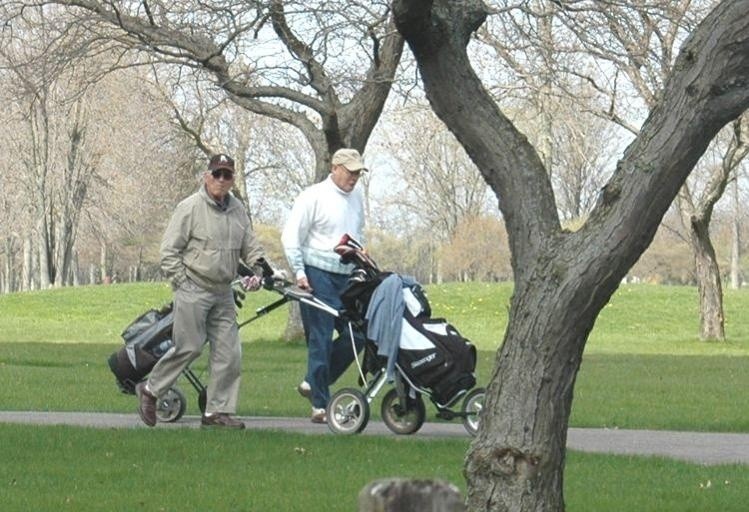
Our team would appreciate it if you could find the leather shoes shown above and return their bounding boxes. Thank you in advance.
[135,380,157,427]
[201,411,246,430]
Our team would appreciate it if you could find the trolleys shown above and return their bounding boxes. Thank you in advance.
[106,270,300,428]
[326,237,491,439]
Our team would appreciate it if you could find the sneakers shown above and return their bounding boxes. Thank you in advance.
[297,385,313,403]
[312,413,328,424]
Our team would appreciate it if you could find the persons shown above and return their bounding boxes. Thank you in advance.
[281,147,370,424]
[135,153,289,431]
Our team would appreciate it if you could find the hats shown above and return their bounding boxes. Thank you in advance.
[208,154,235,173]
[331,148,368,172]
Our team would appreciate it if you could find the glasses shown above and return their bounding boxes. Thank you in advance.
[212,172,233,180]
[339,166,365,177]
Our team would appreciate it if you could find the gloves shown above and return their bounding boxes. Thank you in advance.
[273,268,289,282]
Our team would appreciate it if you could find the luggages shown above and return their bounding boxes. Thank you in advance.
[108,288,246,395]
[339,272,477,408]
